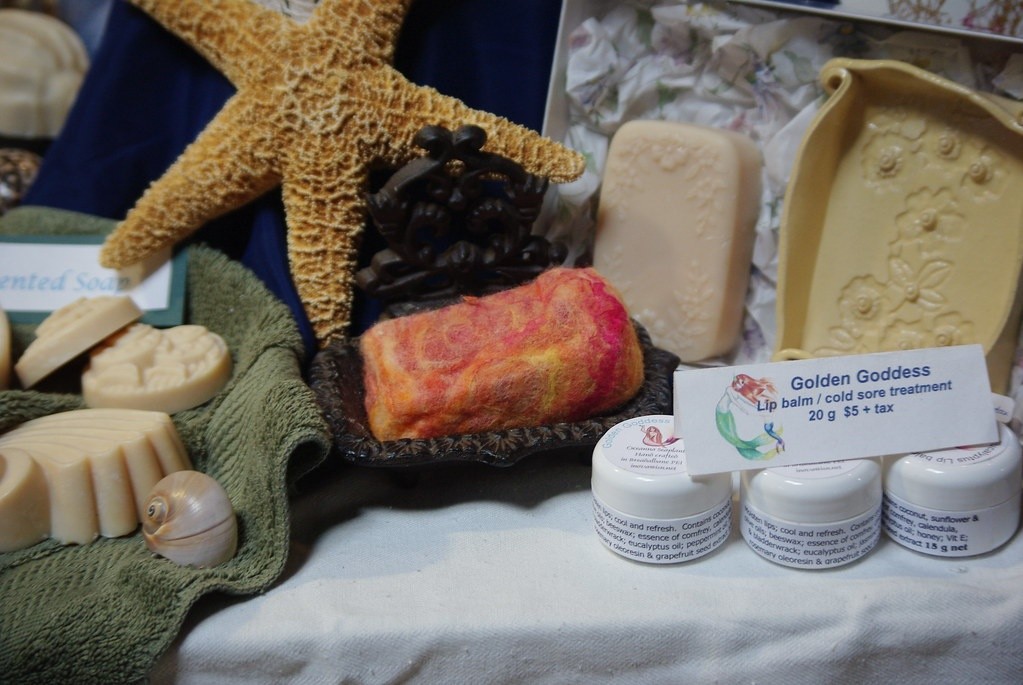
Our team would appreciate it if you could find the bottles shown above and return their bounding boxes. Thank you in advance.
[590,414,733,564]
[739,458,883,570]
[876,420,1023,558]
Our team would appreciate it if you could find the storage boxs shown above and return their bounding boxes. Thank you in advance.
[529,0,1023,442]
